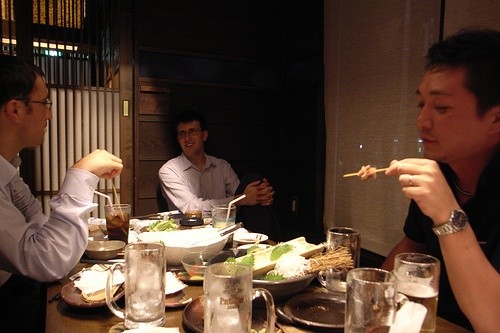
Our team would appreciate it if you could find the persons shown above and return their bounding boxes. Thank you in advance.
[157,112,274,214]
[1,53,124,333]
[380,28,500,331]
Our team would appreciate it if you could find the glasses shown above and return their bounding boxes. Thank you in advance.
[178,127,203,139]
[14,97,52,110]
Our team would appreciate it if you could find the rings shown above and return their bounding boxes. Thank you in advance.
[407,176,416,188]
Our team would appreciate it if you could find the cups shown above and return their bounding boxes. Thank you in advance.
[104,204,132,244]
[203,263,275,333]
[210,203,236,242]
[105,242,167,330]
[326,226,361,295]
[344,267,395,333]
[392,252,440,333]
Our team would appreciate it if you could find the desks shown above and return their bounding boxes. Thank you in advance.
[42,225,471,333]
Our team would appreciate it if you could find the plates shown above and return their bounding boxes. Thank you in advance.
[283,293,363,329]
[182,295,204,333]
[236,243,271,251]
[232,232,268,244]
[60,277,124,308]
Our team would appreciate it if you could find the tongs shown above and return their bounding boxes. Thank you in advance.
[191,222,243,246]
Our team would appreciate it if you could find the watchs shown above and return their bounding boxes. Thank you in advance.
[433,210,470,237]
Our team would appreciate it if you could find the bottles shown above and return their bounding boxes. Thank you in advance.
[179,210,204,226]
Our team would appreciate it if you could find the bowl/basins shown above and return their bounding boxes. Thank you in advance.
[135,228,229,268]
[180,251,216,278]
[252,272,315,303]
[85,240,126,260]
